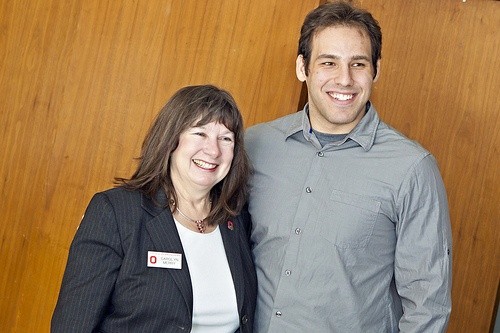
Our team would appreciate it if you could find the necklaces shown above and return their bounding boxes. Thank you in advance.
[170,192,213,233]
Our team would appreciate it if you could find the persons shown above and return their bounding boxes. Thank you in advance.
[50,84,258,333]
[240,2,453,333]
